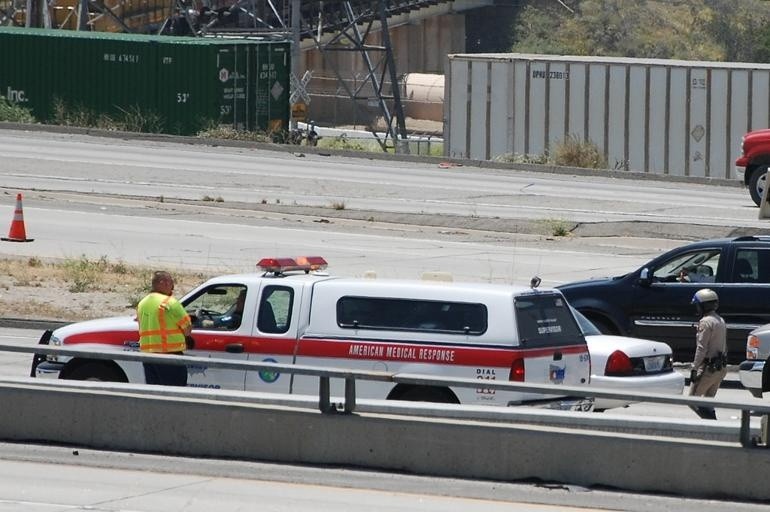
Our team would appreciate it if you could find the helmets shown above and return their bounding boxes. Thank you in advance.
[687,287,720,305]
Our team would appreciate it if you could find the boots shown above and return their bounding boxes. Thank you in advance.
[695,407,717,419]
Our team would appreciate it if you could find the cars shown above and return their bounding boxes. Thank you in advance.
[553,236,770,412]
[735,129,770,207]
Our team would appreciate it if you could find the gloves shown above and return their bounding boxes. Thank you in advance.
[688,369,698,383]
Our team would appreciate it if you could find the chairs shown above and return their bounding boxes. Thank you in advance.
[737,258,754,281]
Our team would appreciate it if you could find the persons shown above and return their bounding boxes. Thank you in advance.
[194,288,247,331]
[160,0,200,36]
[682,288,729,420]
[137,270,193,387]
[678,268,717,283]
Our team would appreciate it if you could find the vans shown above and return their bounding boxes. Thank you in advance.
[30,255,596,407]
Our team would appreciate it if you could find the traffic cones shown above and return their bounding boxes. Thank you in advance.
[0,192,34,242]
